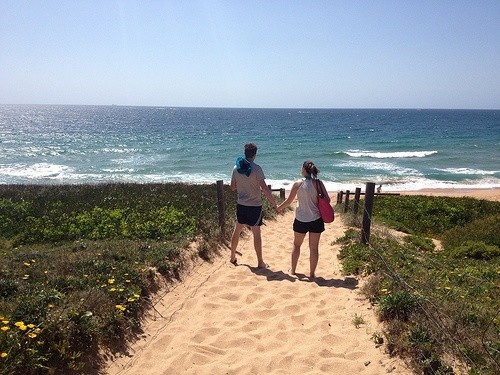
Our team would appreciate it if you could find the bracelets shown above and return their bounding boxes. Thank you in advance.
[274,205,278,209]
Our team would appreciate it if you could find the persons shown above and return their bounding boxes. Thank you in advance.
[376,185,382,196]
[230,143,285,269]
[275,160,331,279]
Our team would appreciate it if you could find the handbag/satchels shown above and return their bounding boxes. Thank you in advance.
[315,179,334,223]
